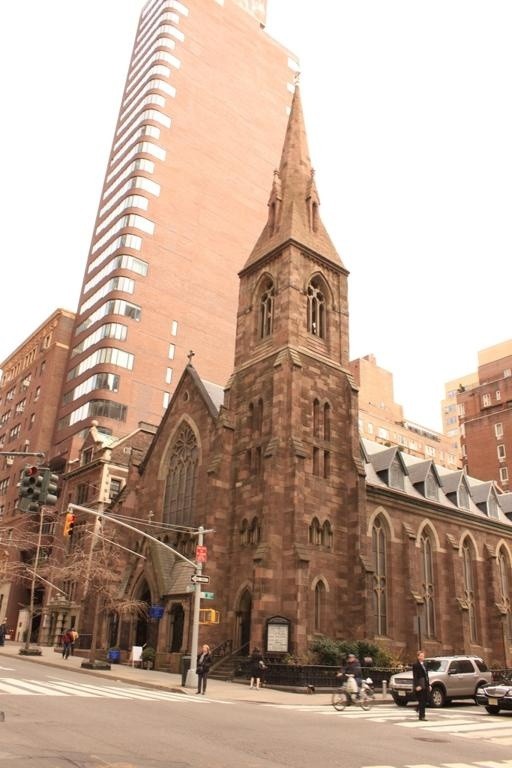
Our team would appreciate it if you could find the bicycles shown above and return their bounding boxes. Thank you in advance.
[331,671,377,710]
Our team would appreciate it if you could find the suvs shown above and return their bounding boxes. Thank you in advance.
[388,653,494,708]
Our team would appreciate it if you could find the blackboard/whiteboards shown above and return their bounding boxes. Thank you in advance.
[134,647,142,660]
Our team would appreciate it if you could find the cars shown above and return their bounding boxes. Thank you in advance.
[473,673,512,713]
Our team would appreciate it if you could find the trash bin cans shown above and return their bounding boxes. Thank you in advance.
[107,648,120,664]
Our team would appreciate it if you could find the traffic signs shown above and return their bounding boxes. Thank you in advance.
[191,574,210,583]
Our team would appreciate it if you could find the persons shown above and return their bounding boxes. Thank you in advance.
[60,627,71,660]
[411,651,433,722]
[335,653,363,707]
[196,644,213,695]
[0,620,7,646]
[70,627,80,657]
[248,646,267,689]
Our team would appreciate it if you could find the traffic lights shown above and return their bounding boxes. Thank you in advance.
[63,514,75,537]
[38,468,59,505]
[15,464,41,512]
[204,608,214,627]
